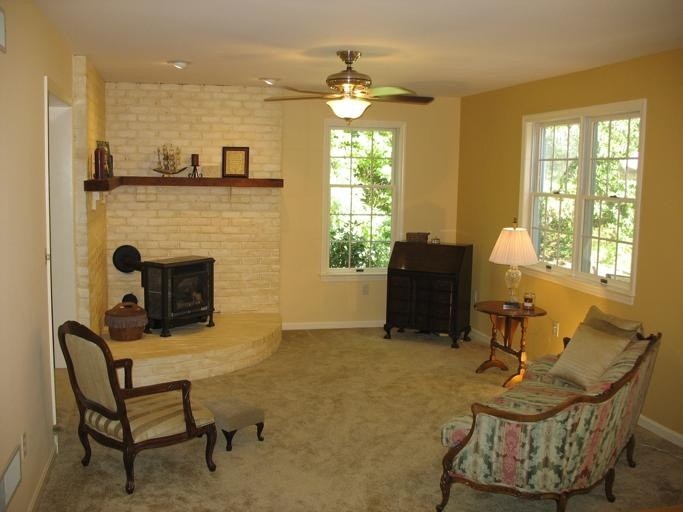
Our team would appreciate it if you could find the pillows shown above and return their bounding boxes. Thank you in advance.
[549,321,637,392]
[583,305,643,331]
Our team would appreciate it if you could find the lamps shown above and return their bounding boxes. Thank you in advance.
[324,98,371,124]
[488,217,540,309]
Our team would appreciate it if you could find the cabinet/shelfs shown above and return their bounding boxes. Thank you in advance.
[141,255,216,337]
[383,241,473,350]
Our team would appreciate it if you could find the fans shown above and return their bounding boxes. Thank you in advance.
[262,50,435,107]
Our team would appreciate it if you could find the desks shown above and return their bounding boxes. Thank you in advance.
[473,301,547,388]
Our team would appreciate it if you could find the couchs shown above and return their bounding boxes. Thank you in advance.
[434,334,660,512]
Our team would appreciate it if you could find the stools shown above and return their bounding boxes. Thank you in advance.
[204,398,265,453]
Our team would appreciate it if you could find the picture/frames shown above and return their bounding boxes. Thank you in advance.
[222,146,249,179]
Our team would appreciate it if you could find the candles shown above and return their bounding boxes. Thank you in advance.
[95,148,105,176]
[191,154,198,166]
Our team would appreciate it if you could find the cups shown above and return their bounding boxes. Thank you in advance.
[524,293,535,310]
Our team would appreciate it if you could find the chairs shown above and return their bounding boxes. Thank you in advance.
[57,319,218,495]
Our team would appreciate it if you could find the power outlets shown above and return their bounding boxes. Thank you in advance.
[551,322,560,337]
[21,433,28,459]
